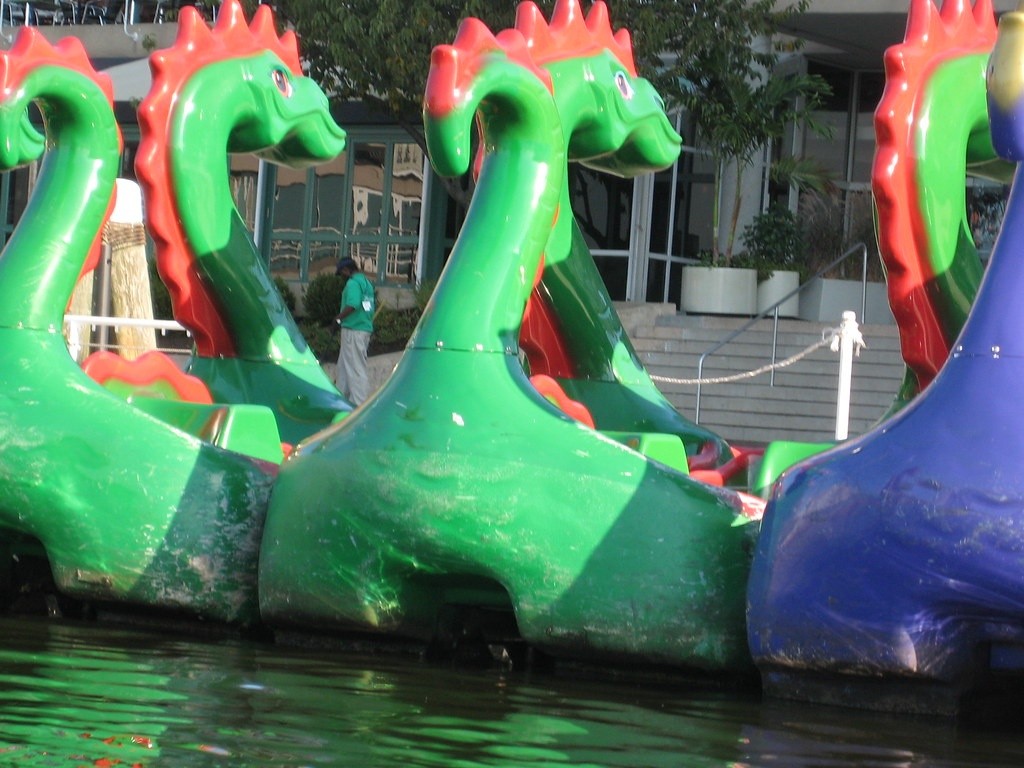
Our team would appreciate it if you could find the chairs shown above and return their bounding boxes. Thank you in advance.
[5,0,125,25]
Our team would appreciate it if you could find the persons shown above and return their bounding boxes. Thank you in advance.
[333,256,375,409]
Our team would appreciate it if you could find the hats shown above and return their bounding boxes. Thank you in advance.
[335,257,355,276]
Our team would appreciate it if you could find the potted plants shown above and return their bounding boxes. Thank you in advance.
[666,56,893,323]
